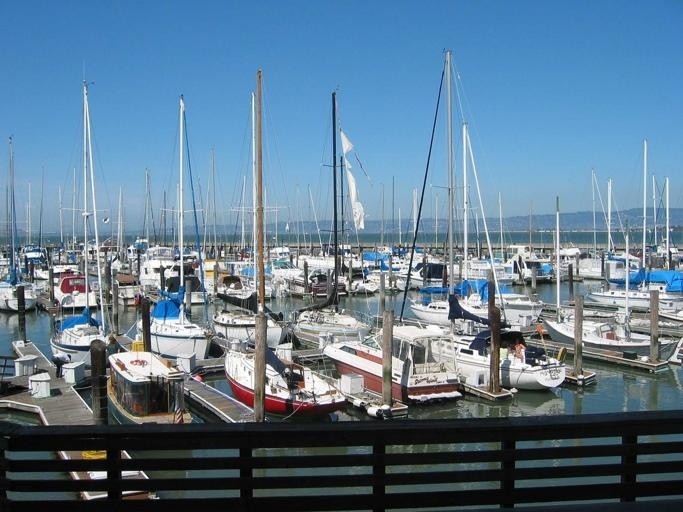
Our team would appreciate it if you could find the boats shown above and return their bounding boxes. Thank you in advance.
[0,48,683,423]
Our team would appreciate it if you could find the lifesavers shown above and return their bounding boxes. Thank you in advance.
[558,348,567,363]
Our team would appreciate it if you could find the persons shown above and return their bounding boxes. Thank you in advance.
[512,338,527,364]
